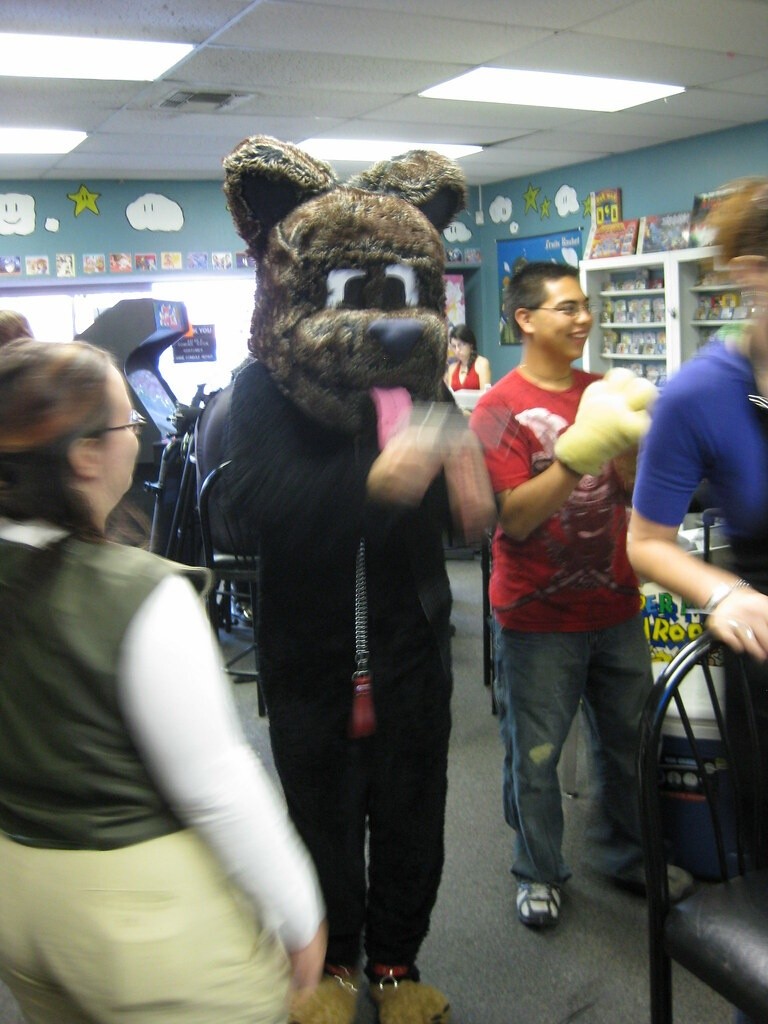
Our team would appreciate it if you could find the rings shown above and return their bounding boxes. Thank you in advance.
[734,623,754,639]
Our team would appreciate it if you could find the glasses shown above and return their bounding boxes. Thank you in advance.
[83,409,145,440]
[519,303,598,317]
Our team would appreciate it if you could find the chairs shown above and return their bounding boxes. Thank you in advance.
[633,631,768,1024]
[199,459,265,717]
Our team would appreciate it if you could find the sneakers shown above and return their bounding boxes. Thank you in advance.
[622,863,697,902]
[515,881,562,928]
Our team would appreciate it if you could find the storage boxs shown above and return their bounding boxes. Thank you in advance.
[663,719,742,880]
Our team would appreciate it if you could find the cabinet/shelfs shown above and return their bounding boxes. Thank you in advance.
[577,245,758,392]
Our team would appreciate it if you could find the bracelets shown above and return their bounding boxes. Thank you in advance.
[703,579,751,615]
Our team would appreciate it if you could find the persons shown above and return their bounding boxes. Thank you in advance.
[470,261,695,929]
[625,170,768,875]
[445,323,493,393]
[0,337,330,1024]
[215,134,503,1018]
[1,310,36,349]
